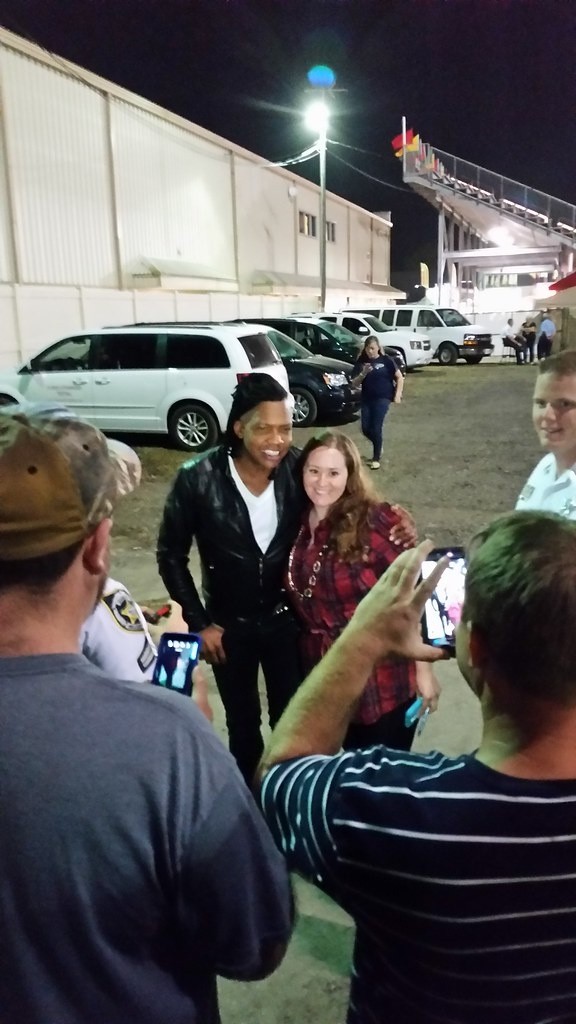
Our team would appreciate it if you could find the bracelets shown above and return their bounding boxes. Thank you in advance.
[358,373,366,379]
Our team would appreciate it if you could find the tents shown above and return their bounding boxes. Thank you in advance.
[533,271,575,309]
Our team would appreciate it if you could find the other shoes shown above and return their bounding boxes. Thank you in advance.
[370,461,380,470]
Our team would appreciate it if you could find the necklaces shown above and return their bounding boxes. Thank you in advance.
[287,524,329,601]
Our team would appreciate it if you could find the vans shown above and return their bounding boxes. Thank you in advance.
[338,306,499,369]
[0,326,294,453]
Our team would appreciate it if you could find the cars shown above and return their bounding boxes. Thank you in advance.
[300,314,437,377]
[229,316,365,427]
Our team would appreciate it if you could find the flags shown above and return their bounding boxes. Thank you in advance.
[390,127,445,182]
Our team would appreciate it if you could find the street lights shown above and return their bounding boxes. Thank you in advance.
[306,102,329,311]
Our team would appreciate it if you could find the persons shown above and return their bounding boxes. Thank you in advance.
[425,587,466,645]
[163,646,181,688]
[155,372,440,779]
[513,350,575,526]
[351,335,404,471]
[0,401,295,1024]
[79,563,205,701]
[250,506,574,1023]
[502,313,556,365]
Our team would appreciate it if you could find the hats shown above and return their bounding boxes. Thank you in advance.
[0,405,142,561]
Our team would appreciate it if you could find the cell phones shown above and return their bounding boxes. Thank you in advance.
[419,546,467,657]
[152,633,202,697]
[405,697,423,727]
